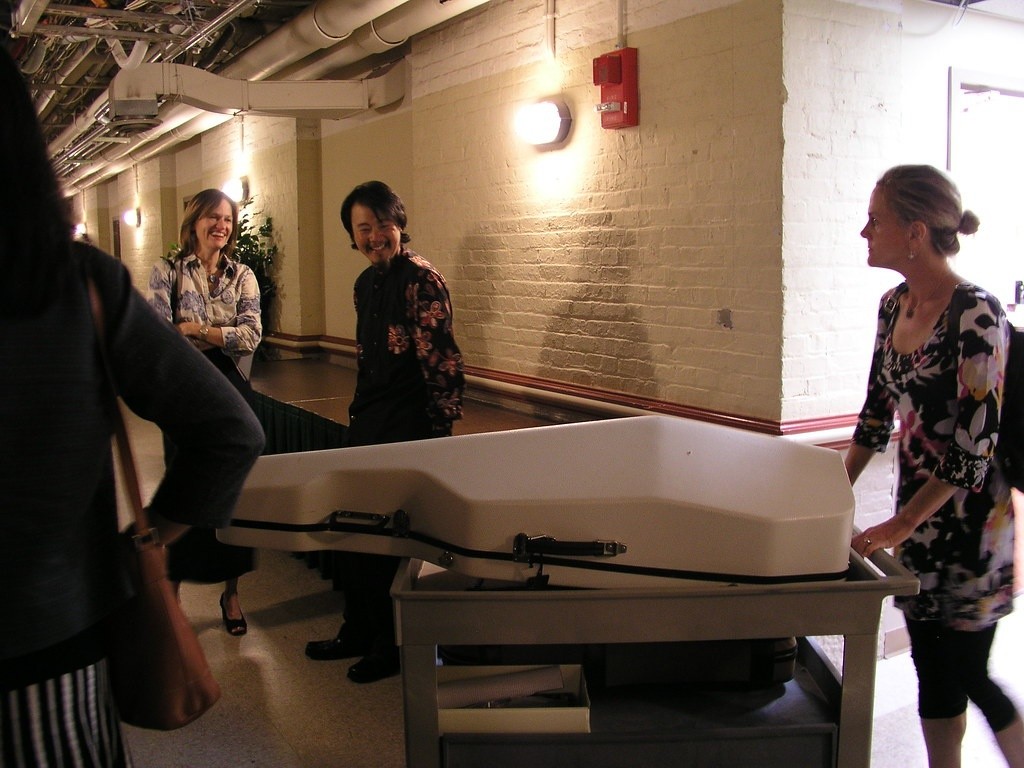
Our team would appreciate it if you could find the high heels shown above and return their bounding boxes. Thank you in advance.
[219,593,249,636]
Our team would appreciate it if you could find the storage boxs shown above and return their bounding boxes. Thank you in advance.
[436,664,594,732]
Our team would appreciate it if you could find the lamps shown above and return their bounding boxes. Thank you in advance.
[124,209,140,227]
[222,175,249,203]
[523,98,571,144]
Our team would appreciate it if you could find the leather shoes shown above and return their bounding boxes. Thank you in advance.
[305,626,363,659]
[346,644,401,682]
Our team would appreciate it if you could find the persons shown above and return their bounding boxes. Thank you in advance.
[0,44,266,768]
[845,165,1024,768]
[305,181,467,683]
[148,188,262,636]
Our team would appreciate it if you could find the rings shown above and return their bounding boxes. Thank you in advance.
[867,539,872,543]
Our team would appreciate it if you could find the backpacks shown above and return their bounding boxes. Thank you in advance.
[878,283,1024,495]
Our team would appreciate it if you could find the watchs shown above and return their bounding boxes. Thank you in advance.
[200,325,208,341]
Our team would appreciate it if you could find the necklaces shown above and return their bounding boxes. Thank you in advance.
[210,274,216,283]
[907,278,943,318]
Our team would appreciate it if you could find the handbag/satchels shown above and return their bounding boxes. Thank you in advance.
[110,533,221,732]
